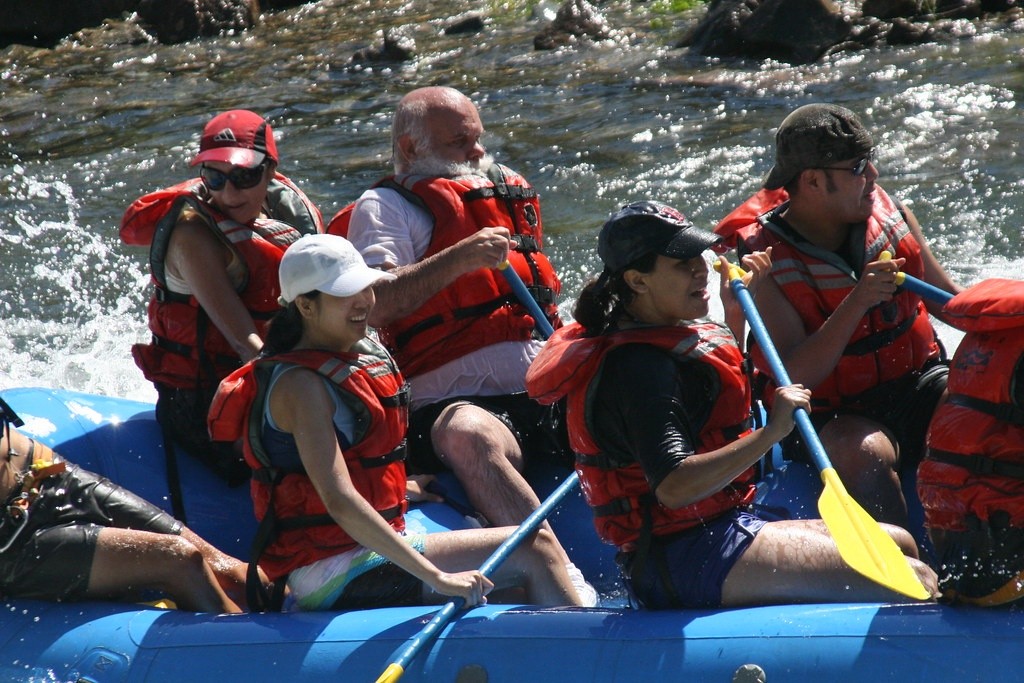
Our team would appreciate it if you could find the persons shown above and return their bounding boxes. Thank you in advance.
[0,399,292,612]
[129,85,1023,604]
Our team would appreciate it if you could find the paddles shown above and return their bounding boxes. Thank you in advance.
[498,255,559,343]
[375,464,589,681]
[884,261,959,305]
[711,250,931,600]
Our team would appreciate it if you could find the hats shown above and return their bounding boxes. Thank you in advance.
[191,110,279,168]
[759,103,874,189]
[597,200,724,277]
[278,233,398,303]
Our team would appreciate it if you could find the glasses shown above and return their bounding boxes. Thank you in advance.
[199,165,265,191]
[808,146,877,176]
